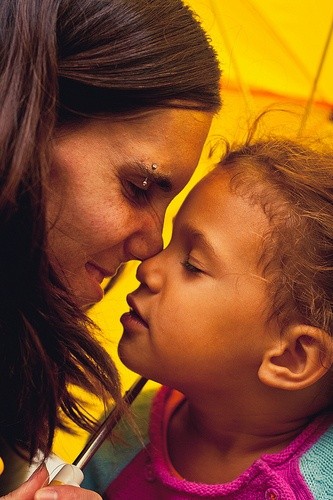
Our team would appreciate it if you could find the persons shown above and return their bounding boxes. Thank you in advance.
[82,105,333,500]
[1,0,221,500]
